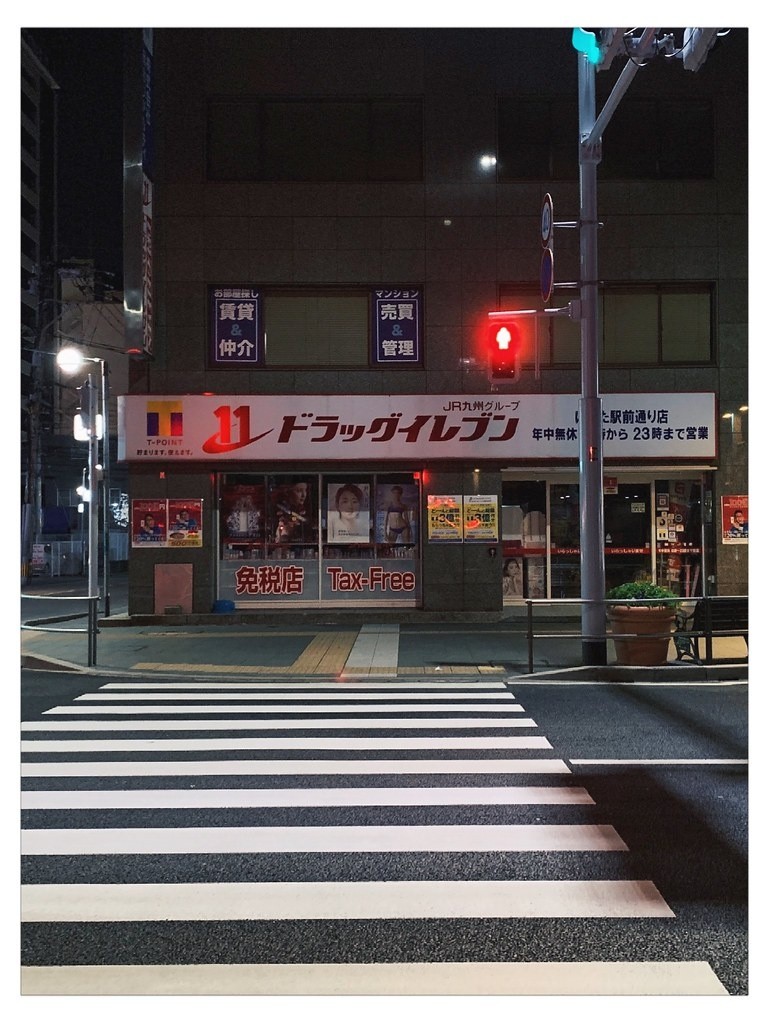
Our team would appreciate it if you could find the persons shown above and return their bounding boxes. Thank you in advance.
[503,558,521,596]
[271,476,309,543]
[328,484,369,541]
[138,514,163,541]
[174,510,198,530]
[728,510,748,537]
[384,486,413,543]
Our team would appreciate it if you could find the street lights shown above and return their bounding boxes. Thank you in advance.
[57,347,111,618]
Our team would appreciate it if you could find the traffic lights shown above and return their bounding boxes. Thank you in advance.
[487,319,520,385]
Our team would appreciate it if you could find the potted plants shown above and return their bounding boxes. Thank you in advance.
[606,581,682,665]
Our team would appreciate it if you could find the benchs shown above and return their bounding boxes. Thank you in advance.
[673,595,750,666]
